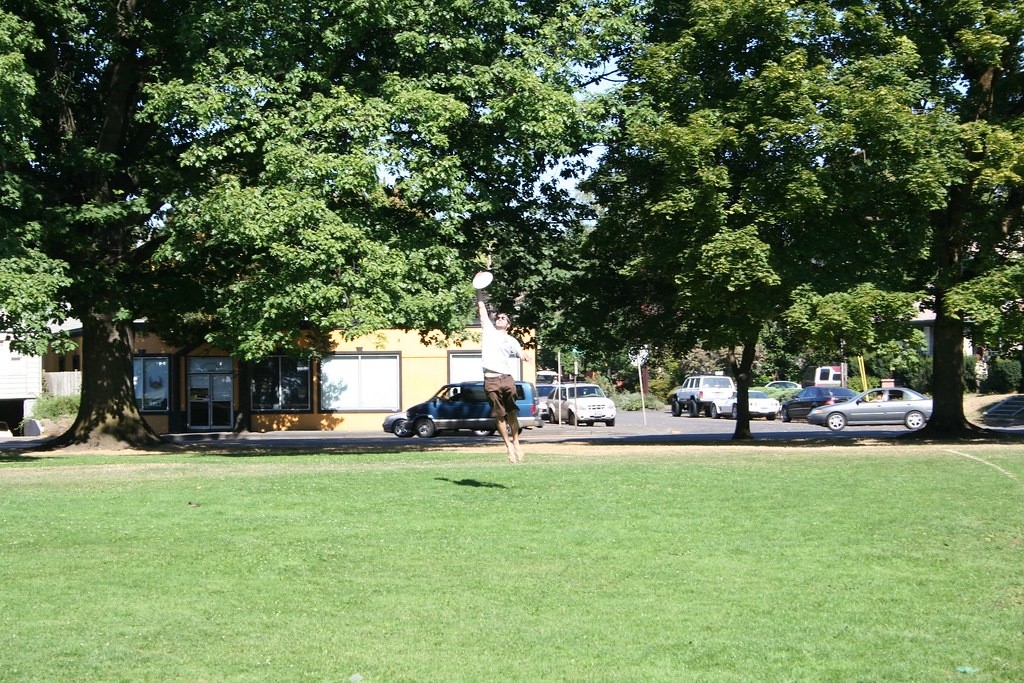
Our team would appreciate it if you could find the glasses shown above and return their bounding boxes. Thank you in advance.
[495,316,511,321]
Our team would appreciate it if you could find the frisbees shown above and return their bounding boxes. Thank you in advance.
[473,271,493,289]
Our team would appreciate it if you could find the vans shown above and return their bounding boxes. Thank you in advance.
[803,366,842,387]
[406,381,543,438]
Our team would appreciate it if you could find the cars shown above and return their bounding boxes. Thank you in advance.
[710,391,781,421]
[536,384,559,415]
[782,386,857,422]
[765,381,802,390]
[806,387,934,430]
[382,410,415,438]
[546,383,616,427]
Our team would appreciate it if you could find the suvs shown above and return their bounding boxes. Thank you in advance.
[671,376,735,417]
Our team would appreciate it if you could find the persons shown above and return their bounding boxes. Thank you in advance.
[443,388,459,400]
[563,392,566,399]
[475,271,530,464]
[974,347,988,399]
[868,393,883,402]
